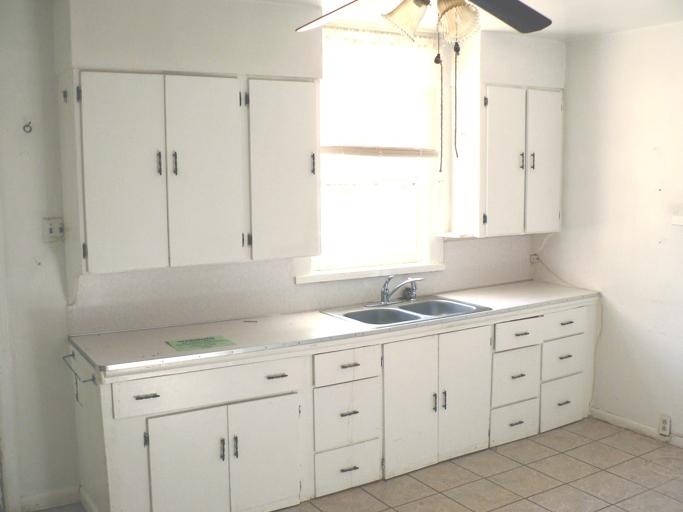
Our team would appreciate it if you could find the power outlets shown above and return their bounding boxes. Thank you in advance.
[531,254,540,264]
[660,416,670,436]
[42,217,65,242]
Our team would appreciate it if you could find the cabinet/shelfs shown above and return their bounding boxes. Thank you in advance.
[313,344,384,499]
[486,86,565,238]
[246,74,323,260]
[492,314,541,444]
[79,67,250,267]
[97,356,309,507]
[541,304,596,430]
[381,309,491,480]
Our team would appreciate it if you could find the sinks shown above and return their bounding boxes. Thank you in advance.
[397,296,493,318]
[344,307,422,326]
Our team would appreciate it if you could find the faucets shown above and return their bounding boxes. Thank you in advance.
[404,277,417,302]
[363,274,426,309]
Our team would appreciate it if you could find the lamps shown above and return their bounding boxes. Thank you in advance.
[387,0,479,54]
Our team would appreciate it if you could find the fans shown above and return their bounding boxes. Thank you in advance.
[295,0,552,36]
